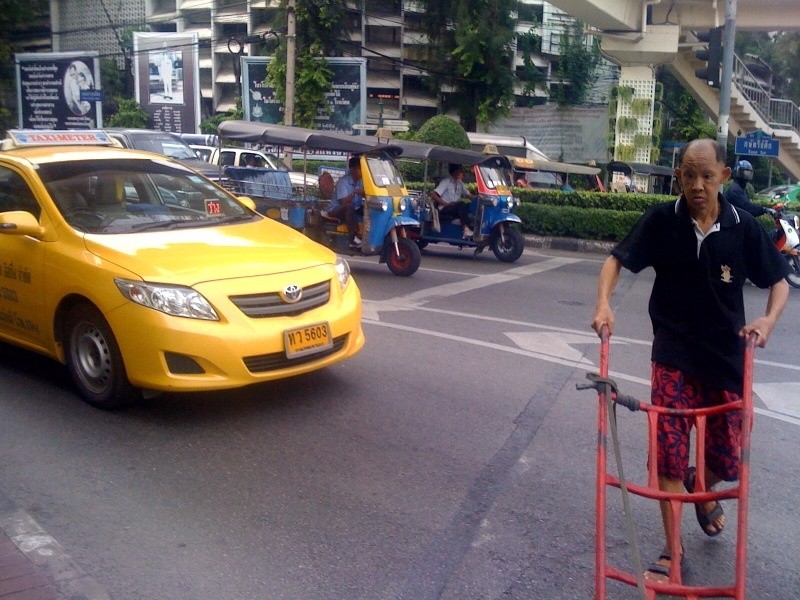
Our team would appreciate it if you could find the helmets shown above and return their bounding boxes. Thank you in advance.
[732,160,754,189]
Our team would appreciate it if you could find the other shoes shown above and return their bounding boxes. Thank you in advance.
[463,227,473,239]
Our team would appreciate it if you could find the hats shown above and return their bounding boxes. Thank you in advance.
[447,163,463,176]
[349,157,361,168]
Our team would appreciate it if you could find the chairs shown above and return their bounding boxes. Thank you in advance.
[52,173,134,219]
[319,172,341,220]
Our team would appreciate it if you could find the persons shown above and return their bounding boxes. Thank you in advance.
[517,173,531,188]
[588,139,791,584]
[245,156,258,168]
[65,65,91,115]
[428,163,476,239]
[725,160,777,287]
[328,157,365,248]
[160,53,183,97]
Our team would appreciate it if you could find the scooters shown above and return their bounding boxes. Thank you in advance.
[504,155,606,192]
[217,120,421,277]
[607,161,676,196]
[766,194,800,289]
[352,135,524,263]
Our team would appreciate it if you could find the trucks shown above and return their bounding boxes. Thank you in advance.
[464,131,563,188]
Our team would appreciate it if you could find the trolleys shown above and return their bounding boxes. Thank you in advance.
[575,324,758,600]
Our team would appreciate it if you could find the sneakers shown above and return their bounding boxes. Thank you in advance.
[349,235,366,248]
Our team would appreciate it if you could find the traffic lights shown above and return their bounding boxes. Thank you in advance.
[695,26,721,88]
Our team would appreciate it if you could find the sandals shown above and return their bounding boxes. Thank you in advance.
[683,467,725,536]
[643,542,685,585]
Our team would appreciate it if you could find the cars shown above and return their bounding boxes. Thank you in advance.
[0,129,365,411]
[755,184,800,202]
[103,129,320,191]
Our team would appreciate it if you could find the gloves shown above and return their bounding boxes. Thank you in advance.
[765,207,778,218]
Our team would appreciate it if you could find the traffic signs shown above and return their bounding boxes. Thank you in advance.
[352,113,410,134]
